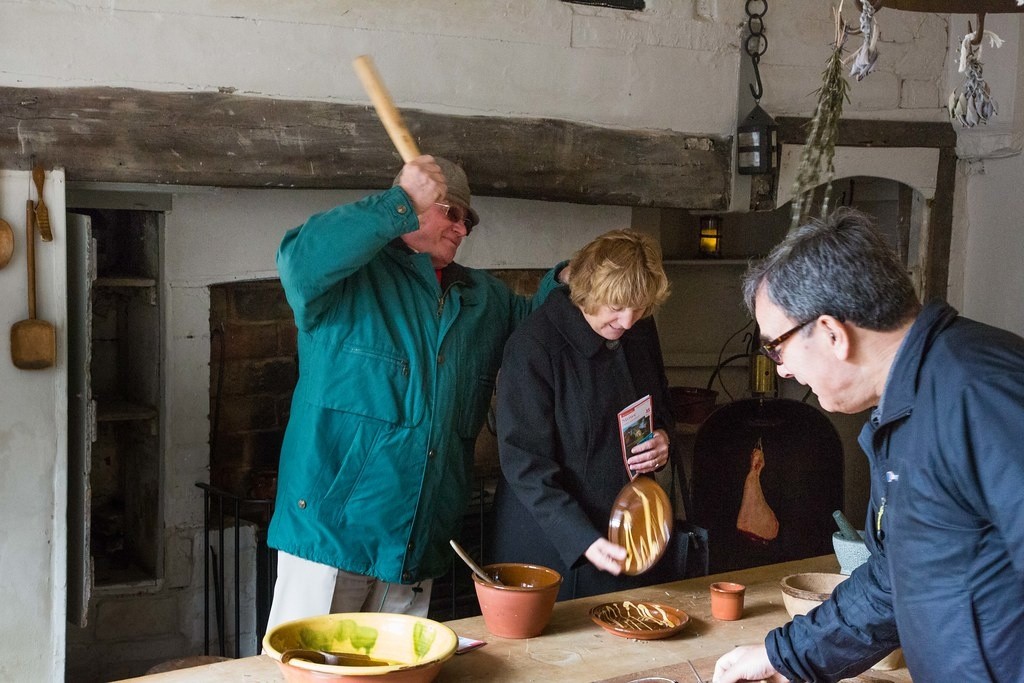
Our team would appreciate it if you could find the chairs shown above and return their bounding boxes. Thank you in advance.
[687,395,846,577]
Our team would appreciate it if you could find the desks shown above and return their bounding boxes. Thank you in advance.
[112,550,915,683]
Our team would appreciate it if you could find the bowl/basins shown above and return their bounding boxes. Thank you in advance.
[261,612,460,683]
[778,572,851,619]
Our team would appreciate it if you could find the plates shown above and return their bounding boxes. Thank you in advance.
[608,476,673,577]
[588,601,692,641]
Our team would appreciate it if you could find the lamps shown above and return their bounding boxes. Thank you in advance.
[736,94,780,175]
[699,215,723,259]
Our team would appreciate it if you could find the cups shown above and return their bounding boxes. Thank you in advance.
[710,581,746,622]
[471,563,564,640]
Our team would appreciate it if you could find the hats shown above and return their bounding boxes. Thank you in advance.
[391,156,480,228]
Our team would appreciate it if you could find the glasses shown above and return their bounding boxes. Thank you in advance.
[433,199,473,237]
[759,314,846,365]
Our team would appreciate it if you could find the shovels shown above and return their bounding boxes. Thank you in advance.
[9,200,56,371]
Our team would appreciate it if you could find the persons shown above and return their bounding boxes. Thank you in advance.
[482,228,675,600]
[711,195,1024,683]
[262,155,573,654]
